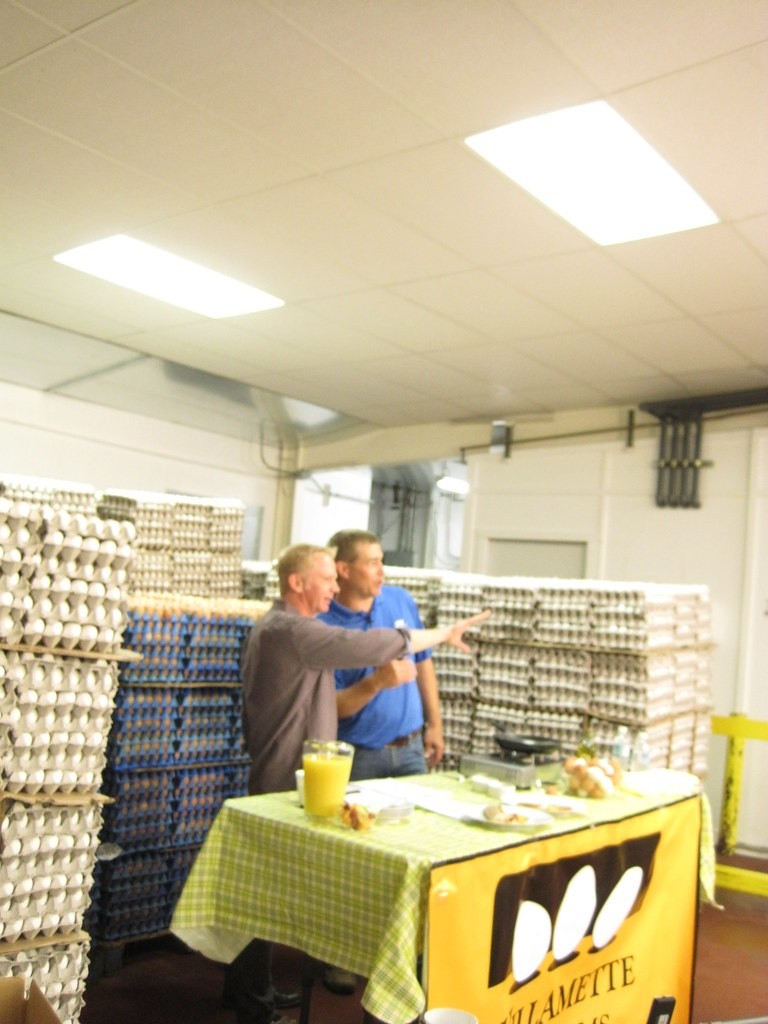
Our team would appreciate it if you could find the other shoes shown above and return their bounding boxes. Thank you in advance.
[222,984,302,1008]
[266,1015,296,1024]
[322,964,353,994]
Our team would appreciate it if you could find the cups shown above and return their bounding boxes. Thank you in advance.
[301,738,355,819]
[295,769,305,808]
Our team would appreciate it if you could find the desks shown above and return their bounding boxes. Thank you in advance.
[170,772,722,1024]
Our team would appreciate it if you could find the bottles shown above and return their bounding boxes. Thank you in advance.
[632,732,651,773]
[577,727,596,759]
[612,726,632,771]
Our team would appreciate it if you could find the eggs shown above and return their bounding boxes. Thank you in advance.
[378,567,710,772]
[0,475,280,1024]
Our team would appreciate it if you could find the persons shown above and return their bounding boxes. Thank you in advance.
[315,528,445,996]
[240,544,492,1008]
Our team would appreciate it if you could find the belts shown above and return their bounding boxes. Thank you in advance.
[387,729,422,748]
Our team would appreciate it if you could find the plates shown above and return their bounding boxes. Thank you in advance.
[514,792,588,816]
[468,803,554,831]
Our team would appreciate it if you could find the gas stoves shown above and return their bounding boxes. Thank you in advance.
[460,751,567,790]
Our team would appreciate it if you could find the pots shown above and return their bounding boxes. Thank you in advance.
[491,720,561,755]
